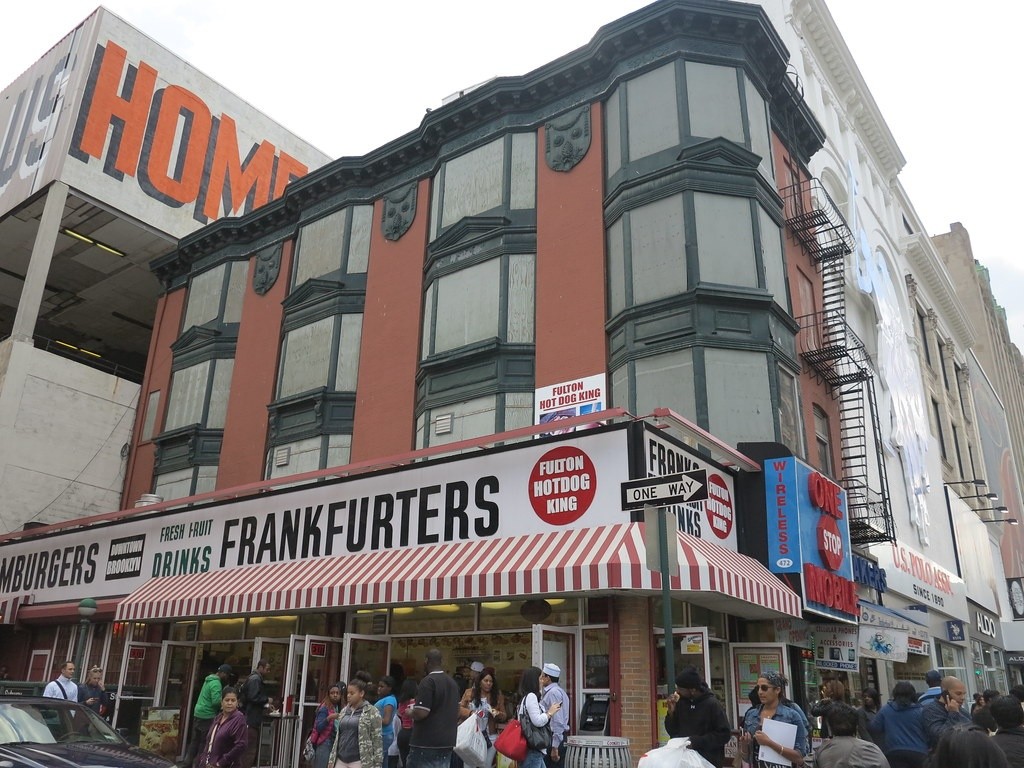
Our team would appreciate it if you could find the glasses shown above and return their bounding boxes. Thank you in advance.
[755,684,777,691]
[93,668,103,674]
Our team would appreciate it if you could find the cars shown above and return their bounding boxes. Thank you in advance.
[0,694,179,768]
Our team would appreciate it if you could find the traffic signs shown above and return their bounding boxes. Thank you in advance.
[621,468,708,512]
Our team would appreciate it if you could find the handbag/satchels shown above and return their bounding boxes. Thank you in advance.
[453,712,488,768]
[519,693,553,751]
[493,696,527,762]
[483,730,492,749]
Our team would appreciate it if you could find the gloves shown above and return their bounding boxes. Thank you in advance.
[687,735,706,748]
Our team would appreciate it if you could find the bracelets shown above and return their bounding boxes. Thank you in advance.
[779,746,784,756]
[548,712,553,717]
[469,710,473,716]
[740,750,750,755]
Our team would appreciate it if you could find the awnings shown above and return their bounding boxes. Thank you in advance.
[113,523,802,623]
[0,592,35,625]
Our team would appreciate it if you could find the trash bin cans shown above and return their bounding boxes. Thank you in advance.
[565,736,631,768]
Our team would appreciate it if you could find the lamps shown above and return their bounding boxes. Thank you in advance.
[983,519,1018,525]
[55,339,102,358]
[943,480,987,487]
[959,493,998,501]
[59,226,127,258]
[971,507,1008,513]
[636,407,761,472]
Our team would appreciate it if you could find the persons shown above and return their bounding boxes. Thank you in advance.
[43,661,108,718]
[0,686,57,744]
[180,659,273,768]
[636,665,1024,768]
[310,649,570,768]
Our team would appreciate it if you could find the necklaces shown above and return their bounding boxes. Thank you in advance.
[763,705,776,718]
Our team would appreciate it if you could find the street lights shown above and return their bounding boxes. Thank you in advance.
[70,599,98,685]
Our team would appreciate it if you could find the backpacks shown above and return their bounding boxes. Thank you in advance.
[234,673,254,714]
[302,729,319,763]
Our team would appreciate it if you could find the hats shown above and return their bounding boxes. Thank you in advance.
[218,663,236,676]
[972,693,980,700]
[543,663,561,678]
[470,661,485,673]
